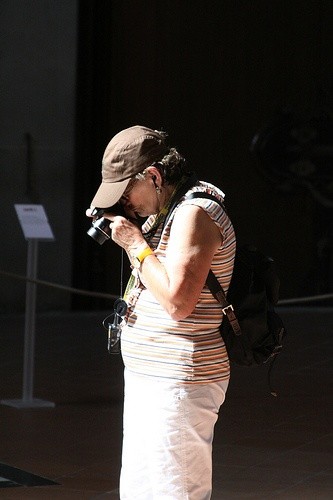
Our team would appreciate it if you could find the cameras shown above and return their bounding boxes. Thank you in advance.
[86,200,140,247]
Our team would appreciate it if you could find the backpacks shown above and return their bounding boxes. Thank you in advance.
[166,189,287,397]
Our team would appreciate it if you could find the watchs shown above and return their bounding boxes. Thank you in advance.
[132,248,155,271]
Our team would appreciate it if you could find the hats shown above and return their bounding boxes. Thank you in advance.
[85,122,167,213]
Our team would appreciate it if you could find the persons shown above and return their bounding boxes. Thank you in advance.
[85,125,237,500]
